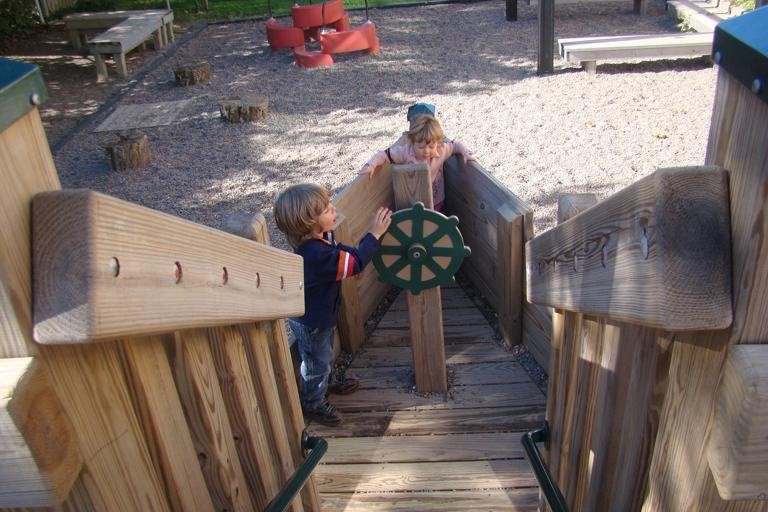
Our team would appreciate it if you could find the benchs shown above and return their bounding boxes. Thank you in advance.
[87,14,164,82]
[67,8,175,51]
[666,0,724,32]
[557,32,715,74]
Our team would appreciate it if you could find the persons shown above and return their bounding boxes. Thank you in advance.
[357,113,484,213]
[274,184,393,428]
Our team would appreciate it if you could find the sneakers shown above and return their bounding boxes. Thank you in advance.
[306,401,342,427]
[329,378,359,394]
